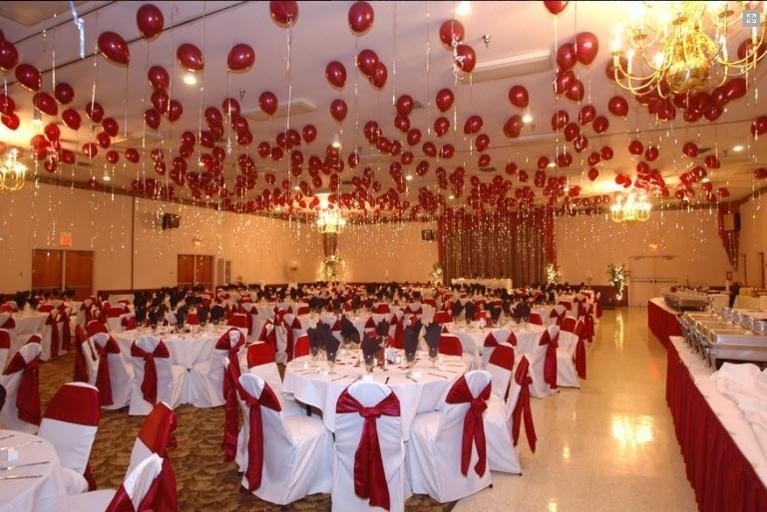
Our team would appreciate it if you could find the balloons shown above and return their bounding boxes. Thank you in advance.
[268,1,298,30]
[437,20,465,48]
[544,1,570,16]
[0,25,766,234]
[348,1,376,36]
[135,4,166,43]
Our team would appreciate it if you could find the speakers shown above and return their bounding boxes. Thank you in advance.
[421,229,432,241]
[724,213,740,231]
[164,213,180,228]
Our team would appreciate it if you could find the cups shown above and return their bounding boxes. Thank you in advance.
[134,316,229,336]
[302,344,444,376]
[453,313,530,331]
[309,306,374,321]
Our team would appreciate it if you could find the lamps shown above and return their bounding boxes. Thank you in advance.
[1,144,30,198]
[34,109,43,123]
[244,97,318,122]
[601,0,765,100]
[112,132,163,150]
[310,204,348,236]
[609,188,649,225]
[459,51,556,88]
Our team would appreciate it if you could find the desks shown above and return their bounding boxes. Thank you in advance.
[645,291,767,511]
